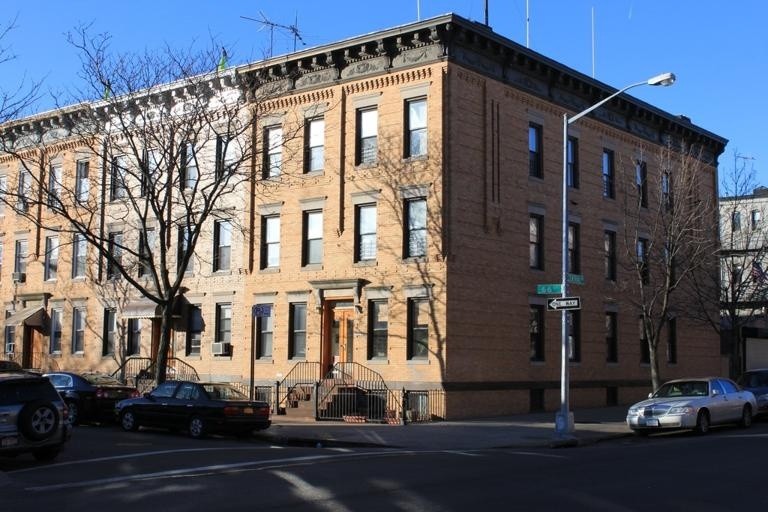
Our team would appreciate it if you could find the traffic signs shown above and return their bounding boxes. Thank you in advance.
[547,296,582,313]
[536,272,585,295]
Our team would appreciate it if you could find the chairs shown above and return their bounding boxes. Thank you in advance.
[670,385,683,396]
[691,384,708,396]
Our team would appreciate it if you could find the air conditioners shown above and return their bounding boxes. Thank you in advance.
[212,343,229,355]
[12,273,25,282]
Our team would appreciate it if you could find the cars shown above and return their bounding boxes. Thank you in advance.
[42,372,271,439]
[626,369,768,435]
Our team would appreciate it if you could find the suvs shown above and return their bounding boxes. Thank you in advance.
[0,361,69,461]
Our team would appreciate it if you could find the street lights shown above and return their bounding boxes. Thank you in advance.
[555,72,677,433]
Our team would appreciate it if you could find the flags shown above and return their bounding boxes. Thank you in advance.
[104,80,111,101]
[219,47,227,72]
